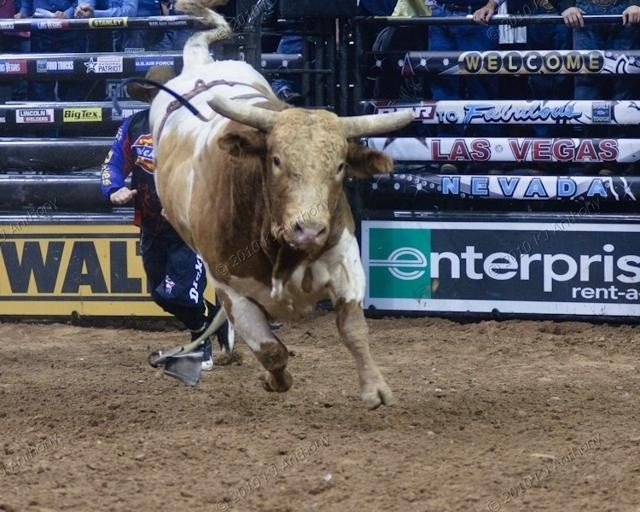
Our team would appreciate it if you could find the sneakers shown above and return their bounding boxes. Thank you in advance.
[217,310,236,353]
[190,323,213,371]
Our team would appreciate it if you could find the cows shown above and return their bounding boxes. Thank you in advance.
[149,0,415,411]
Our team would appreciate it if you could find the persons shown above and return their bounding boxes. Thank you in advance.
[100,64,235,369]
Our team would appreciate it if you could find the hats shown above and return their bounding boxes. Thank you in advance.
[126,65,176,105]
